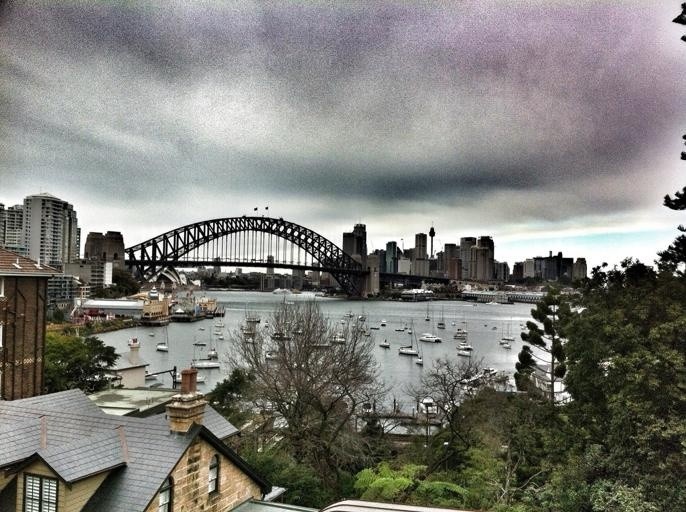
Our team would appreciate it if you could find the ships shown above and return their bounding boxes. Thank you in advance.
[136,283,226,329]
[475,291,514,305]
[397,287,434,302]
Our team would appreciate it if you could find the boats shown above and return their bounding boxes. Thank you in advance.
[362,400,372,416]
[145,329,225,383]
[449,366,508,412]
[418,398,438,418]
